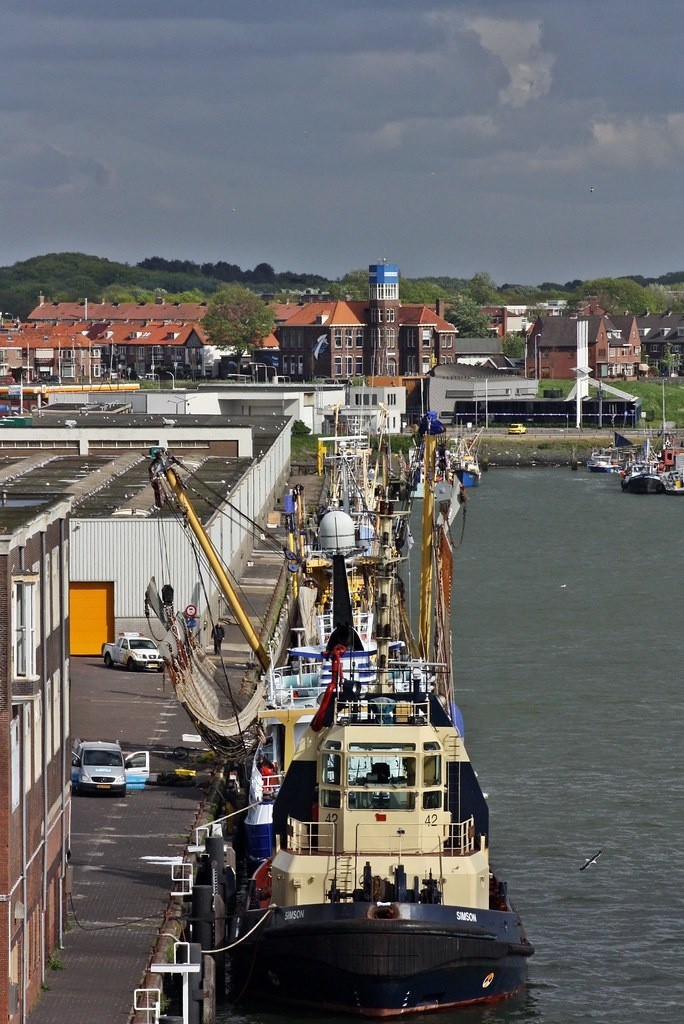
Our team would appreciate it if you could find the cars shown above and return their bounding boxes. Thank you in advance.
[508,424,526,435]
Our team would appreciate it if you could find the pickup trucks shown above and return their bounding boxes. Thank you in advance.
[102,632,164,673]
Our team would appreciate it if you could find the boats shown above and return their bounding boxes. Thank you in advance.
[585,432,667,494]
[232,346,535,1019]
[447,418,486,487]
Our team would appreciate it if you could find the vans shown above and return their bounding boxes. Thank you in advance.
[72,739,150,796]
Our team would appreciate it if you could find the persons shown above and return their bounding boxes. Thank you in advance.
[211,620,225,655]
